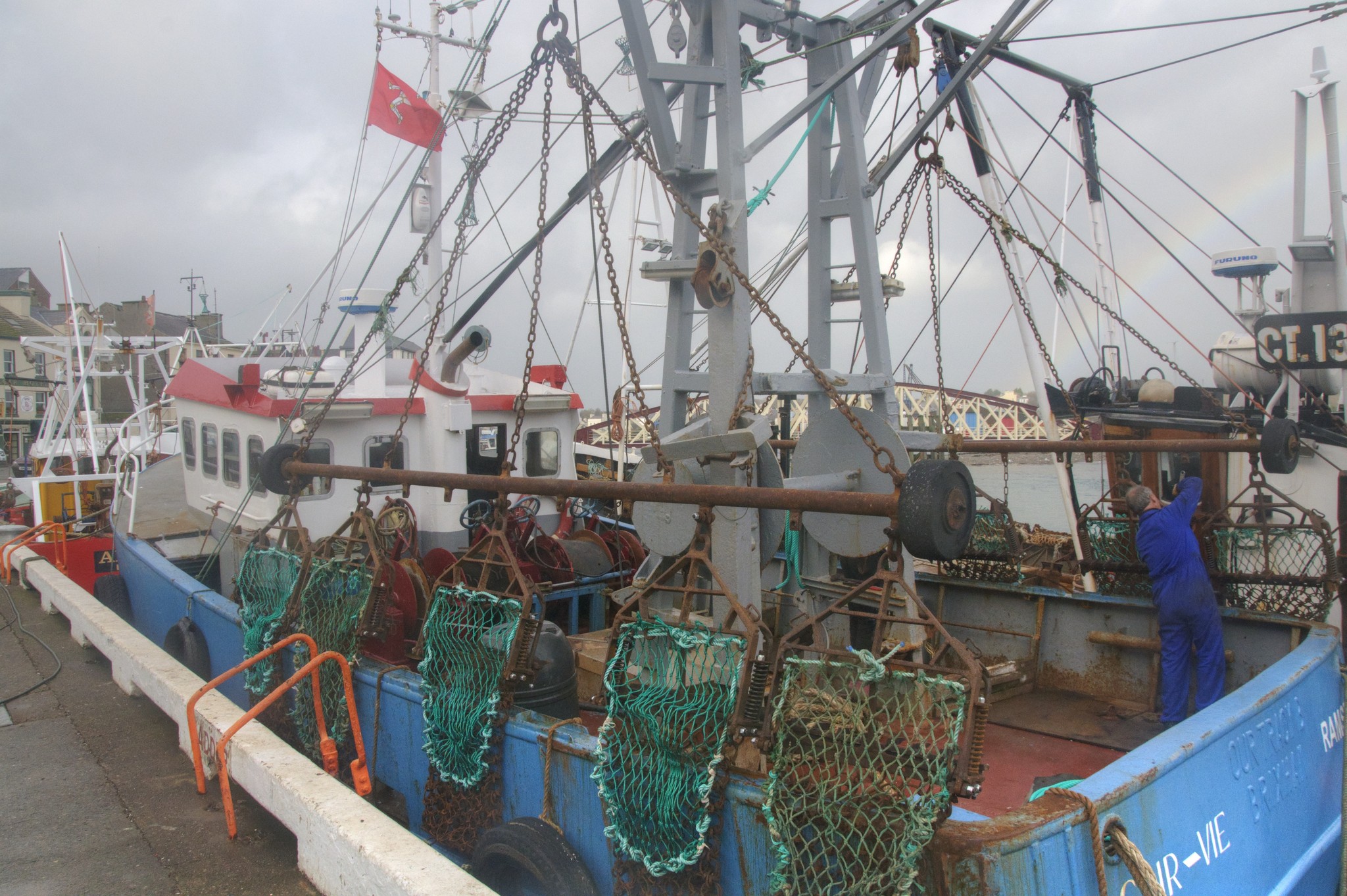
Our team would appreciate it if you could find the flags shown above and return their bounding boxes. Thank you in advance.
[145,294,155,334]
[367,60,446,152]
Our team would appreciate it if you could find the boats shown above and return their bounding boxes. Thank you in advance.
[954,45,1347,624]
[18,229,187,597]
[100,1,1347,896]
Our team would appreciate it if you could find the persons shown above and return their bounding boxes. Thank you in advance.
[1123,478,1228,735]
